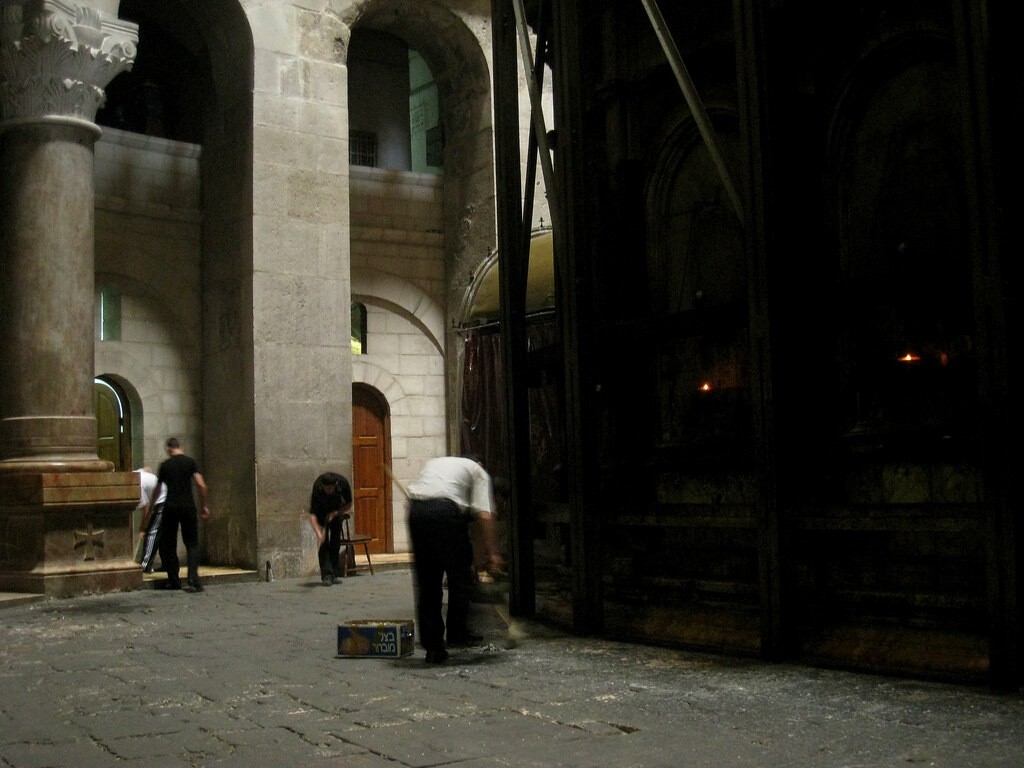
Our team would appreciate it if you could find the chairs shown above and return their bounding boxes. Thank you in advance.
[332,514,376,577]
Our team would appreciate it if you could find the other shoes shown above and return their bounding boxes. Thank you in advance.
[425,650,450,663]
[331,574,342,584]
[189,579,204,592]
[446,631,484,643]
[166,583,182,589]
[322,574,332,586]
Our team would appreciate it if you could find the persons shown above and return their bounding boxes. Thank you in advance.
[407,456,501,663]
[309,472,352,585]
[144,438,209,592]
[132,466,168,573]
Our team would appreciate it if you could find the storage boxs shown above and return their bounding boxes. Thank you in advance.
[337,619,414,658]
